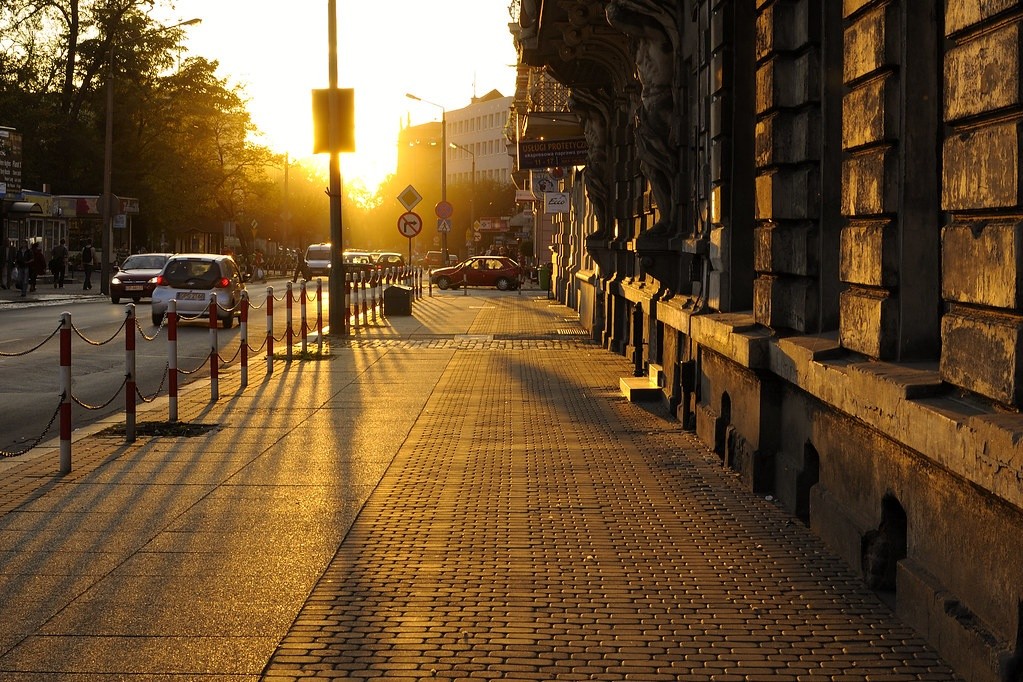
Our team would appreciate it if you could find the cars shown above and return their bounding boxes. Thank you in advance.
[151,253,251,328]
[432,255,525,291]
[404,250,459,270]
[342,247,404,283]
[110,252,174,305]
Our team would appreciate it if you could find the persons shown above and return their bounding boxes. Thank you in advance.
[477,260,483,269]
[52,239,68,288]
[567,86,609,240]
[249,249,267,283]
[291,249,309,283]
[222,245,230,255]
[480,245,506,256]
[185,263,194,276]
[606,3,674,237]
[0,238,46,296]
[117,243,130,266]
[81,239,97,290]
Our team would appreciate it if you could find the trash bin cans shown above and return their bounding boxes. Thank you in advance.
[537,262,553,291]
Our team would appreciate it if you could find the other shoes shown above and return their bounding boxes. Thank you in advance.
[30,284,36,292]
[21,293,26,297]
[54,282,65,289]
[83,285,93,290]
[291,279,297,283]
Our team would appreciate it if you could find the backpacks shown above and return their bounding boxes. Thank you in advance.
[83,246,94,262]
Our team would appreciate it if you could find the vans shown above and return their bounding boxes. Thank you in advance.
[305,242,332,279]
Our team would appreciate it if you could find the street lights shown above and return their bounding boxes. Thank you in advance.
[449,141,476,255]
[405,92,447,269]
[100,17,202,297]
